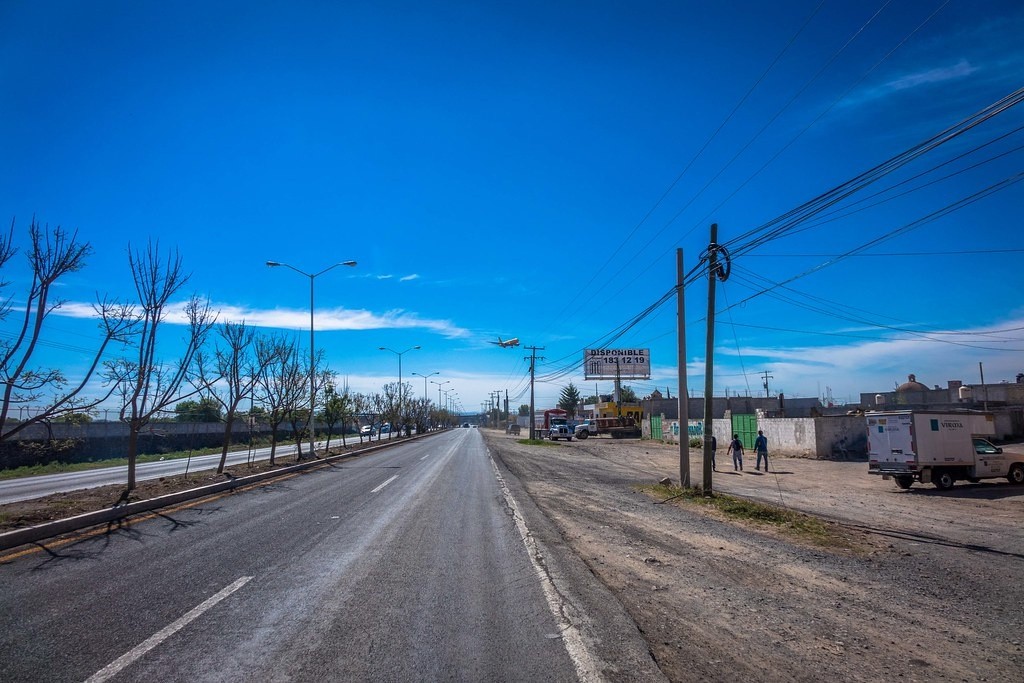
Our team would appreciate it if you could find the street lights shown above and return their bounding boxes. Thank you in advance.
[430,380,449,411]
[379,346,421,436]
[412,372,440,418]
[445,394,463,412]
[267,260,358,455]
[440,389,453,410]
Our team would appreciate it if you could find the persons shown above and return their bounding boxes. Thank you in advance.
[726,434,745,471]
[753,430,769,472]
[711,432,717,472]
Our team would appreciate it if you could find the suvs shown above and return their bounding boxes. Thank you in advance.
[508,424,520,435]
[381,423,390,433]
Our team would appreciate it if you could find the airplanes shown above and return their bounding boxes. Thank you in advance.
[488,336,520,349]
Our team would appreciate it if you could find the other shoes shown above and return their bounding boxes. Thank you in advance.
[740,468,743,470]
[734,468,737,471]
[754,467,759,470]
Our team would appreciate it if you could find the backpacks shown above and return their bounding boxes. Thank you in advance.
[733,439,740,451]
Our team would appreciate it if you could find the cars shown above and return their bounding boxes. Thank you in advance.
[360,425,376,437]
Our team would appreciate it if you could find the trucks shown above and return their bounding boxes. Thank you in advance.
[575,417,636,439]
[545,408,572,441]
[864,410,1024,489]
